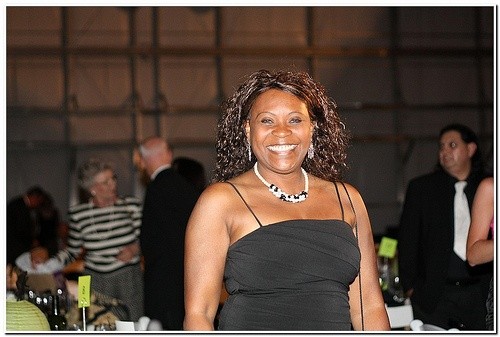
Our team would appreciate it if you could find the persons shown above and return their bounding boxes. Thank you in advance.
[7,187,46,265]
[466,177,494,330]
[183,67,392,331]
[132,136,199,331]
[36,159,143,323]
[34,192,62,258]
[397,124,491,331]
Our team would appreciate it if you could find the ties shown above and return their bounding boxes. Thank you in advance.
[453,181,472,261]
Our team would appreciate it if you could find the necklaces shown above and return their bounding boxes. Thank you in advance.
[88,198,115,240]
[254,162,308,203]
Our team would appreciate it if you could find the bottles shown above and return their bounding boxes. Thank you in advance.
[48,295,67,331]
